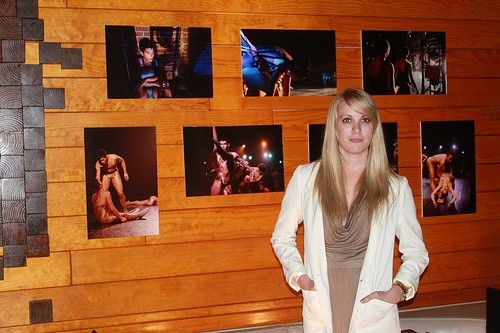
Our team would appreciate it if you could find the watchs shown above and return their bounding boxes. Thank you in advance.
[392,280,409,302]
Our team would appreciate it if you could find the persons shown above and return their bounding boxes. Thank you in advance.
[209,126,270,196]
[271,44,311,97]
[269,86,430,333]
[363,37,420,95]
[131,38,173,99]
[422,151,457,208]
[89,148,158,223]
